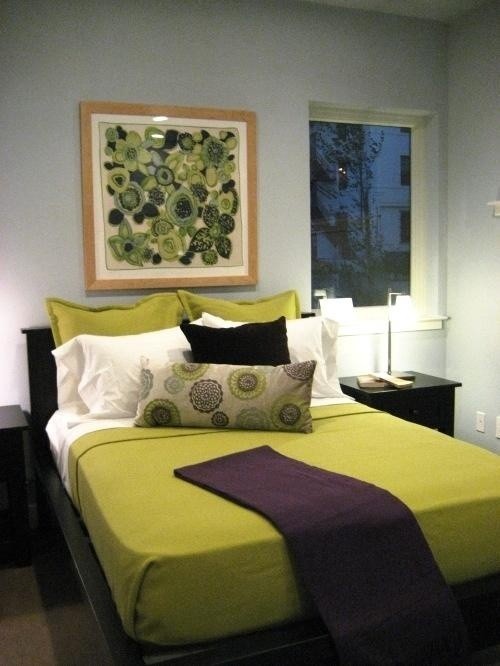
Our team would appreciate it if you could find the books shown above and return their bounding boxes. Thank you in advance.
[357,376,385,388]
[372,371,414,388]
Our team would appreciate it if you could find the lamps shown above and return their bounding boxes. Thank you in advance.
[375,293,415,379]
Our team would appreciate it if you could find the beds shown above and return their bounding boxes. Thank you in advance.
[21,326,500,665]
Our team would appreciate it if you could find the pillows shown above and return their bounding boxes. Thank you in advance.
[180,316,292,366]
[202,311,344,398]
[44,292,189,348]
[176,290,302,322]
[134,355,318,433]
[50,318,205,430]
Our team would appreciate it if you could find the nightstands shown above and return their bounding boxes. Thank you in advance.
[339,371,462,438]
[0,405,31,568]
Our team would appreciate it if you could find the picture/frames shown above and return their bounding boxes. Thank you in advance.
[78,102,258,290]
